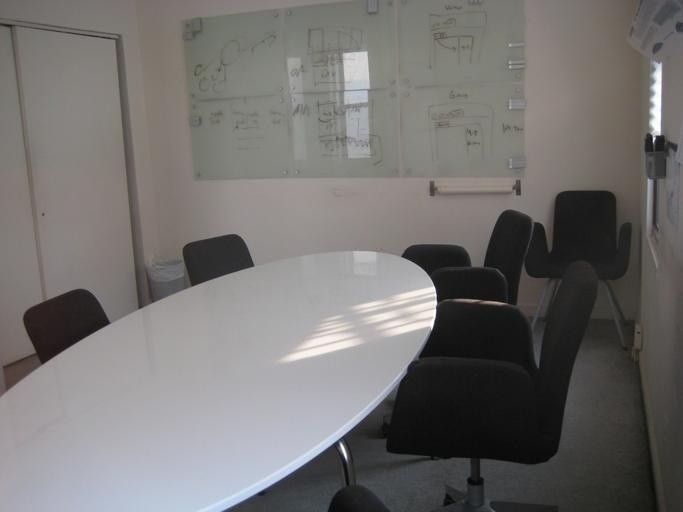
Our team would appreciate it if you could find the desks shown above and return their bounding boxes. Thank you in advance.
[1,251,439,512]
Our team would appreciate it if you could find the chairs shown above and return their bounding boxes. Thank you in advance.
[403,207,534,303]
[183,234,254,286]
[525,190,632,350]
[388,260,598,512]
[23,289,110,365]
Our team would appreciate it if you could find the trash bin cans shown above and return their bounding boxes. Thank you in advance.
[146,259,187,302]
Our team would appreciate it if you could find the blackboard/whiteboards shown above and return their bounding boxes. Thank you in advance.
[182,1,527,181]
